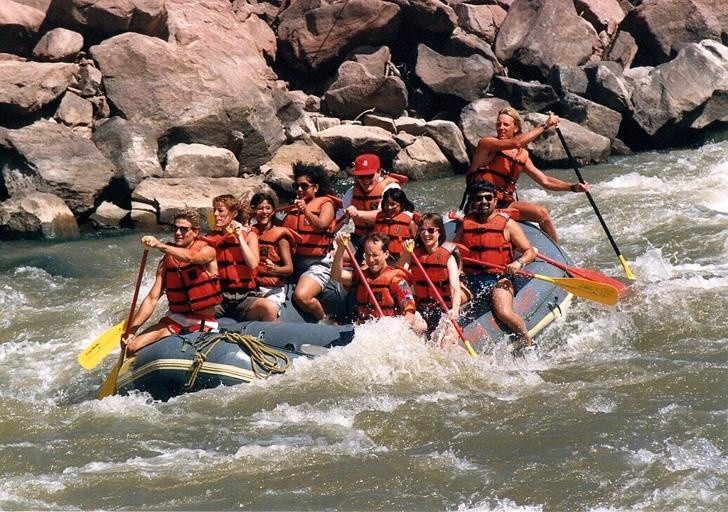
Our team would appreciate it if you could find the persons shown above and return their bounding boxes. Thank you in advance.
[371,188,423,270]
[204,194,277,322]
[332,155,403,260]
[331,232,417,329]
[397,212,469,355]
[454,182,538,352]
[272,169,342,326]
[244,195,293,321]
[465,106,590,243]
[120,212,220,358]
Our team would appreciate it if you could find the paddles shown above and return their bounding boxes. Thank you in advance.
[449,211,627,296]
[258,261,270,268]
[341,237,386,321]
[549,111,636,282]
[459,256,618,307]
[335,209,352,226]
[76,225,237,372]
[206,202,307,231]
[97,238,152,400]
[406,244,475,357]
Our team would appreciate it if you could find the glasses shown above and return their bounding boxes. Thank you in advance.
[475,195,495,201]
[170,224,195,233]
[362,252,385,259]
[292,182,315,190]
[384,203,403,209]
[419,226,441,234]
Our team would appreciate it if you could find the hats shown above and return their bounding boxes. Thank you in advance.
[352,154,380,175]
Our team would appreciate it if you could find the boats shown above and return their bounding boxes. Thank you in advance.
[112,216,576,403]
